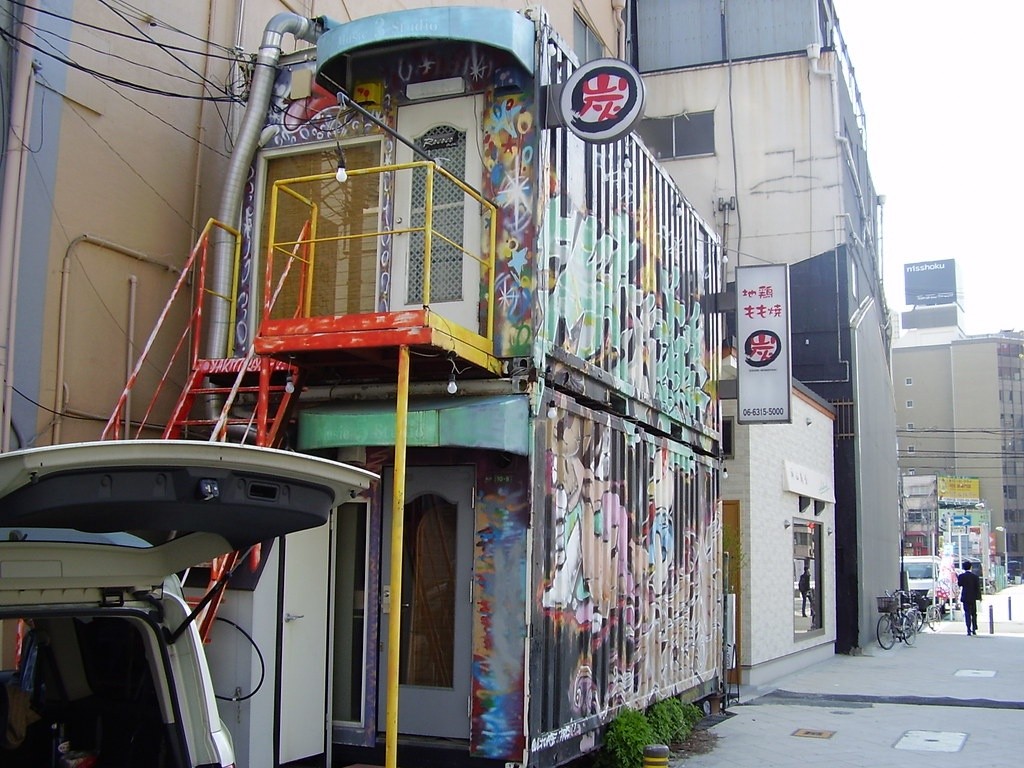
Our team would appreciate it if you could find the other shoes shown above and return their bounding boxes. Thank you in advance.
[802,615,807,617]
[967,631,976,636]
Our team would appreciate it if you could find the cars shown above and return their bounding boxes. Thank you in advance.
[900,555,947,619]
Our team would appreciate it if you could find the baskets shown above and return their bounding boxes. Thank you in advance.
[876,596,898,613]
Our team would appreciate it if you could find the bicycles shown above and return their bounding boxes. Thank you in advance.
[876,588,942,651]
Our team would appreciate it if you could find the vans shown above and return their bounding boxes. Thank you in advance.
[1,436,382,768]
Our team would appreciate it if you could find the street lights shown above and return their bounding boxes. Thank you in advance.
[994,525,1008,584]
[975,501,987,593]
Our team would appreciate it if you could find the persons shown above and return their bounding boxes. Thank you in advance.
[958,561,982,636]
[798,567,815,617]
